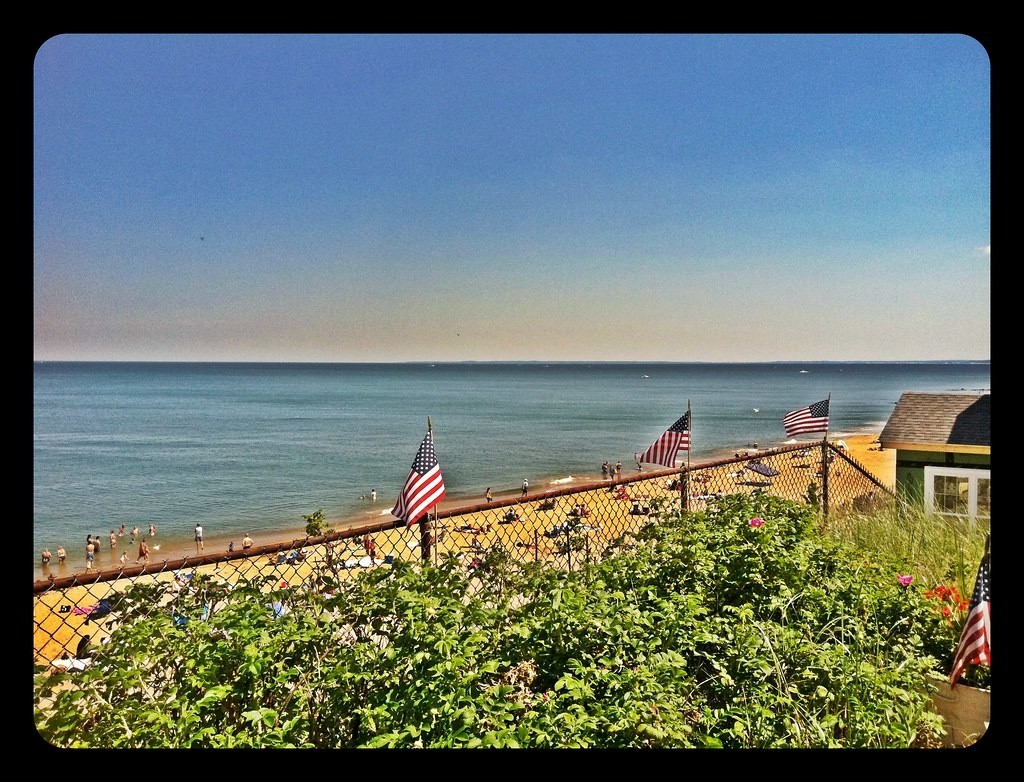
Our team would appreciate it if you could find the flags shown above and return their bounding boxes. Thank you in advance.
[641,411,692,468]
[390,431,452,538]
[782,400,829,437]
[949,553,991,688]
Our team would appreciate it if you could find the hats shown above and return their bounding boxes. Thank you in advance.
[524,479,528,481]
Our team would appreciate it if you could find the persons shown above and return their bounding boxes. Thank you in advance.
[39,443,883,671]
